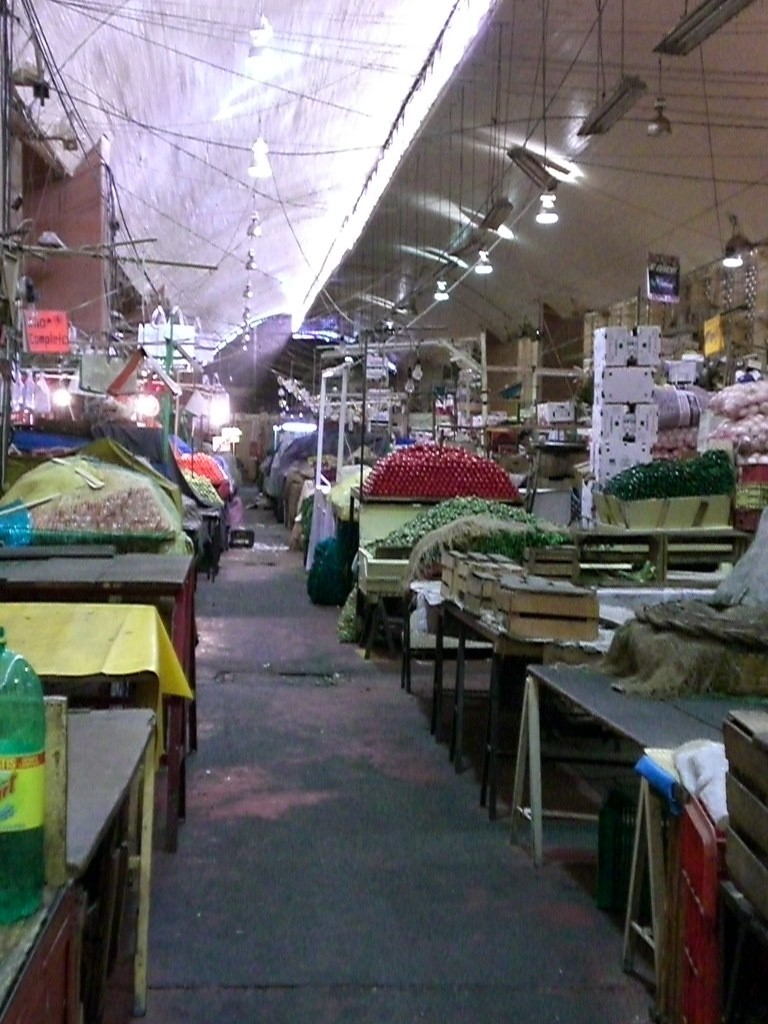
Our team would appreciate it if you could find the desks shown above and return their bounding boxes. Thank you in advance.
[0,466,768,1024]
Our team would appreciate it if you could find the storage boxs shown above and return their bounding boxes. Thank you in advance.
[581,325,661,530]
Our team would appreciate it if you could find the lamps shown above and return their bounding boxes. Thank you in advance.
[451,75,486,259]
[650,0,754,56]
[241,306,252,349]
[247,136,273,178]
[480,21,514,231]
[723,214,758,269]
[577,0,646,136]
[506,147,560,193]
[243,280,253,299]
[434,113,449,302]
[647,55,674,140]
[245,249,258,271]
[475,24,505,275]
[247,210,262,237]
[536,0,560,225]
[433,131,457,281]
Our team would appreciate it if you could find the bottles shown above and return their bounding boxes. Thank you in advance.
[435,394,444,425]
[0,625,47,924]
[446,394,454,424]
